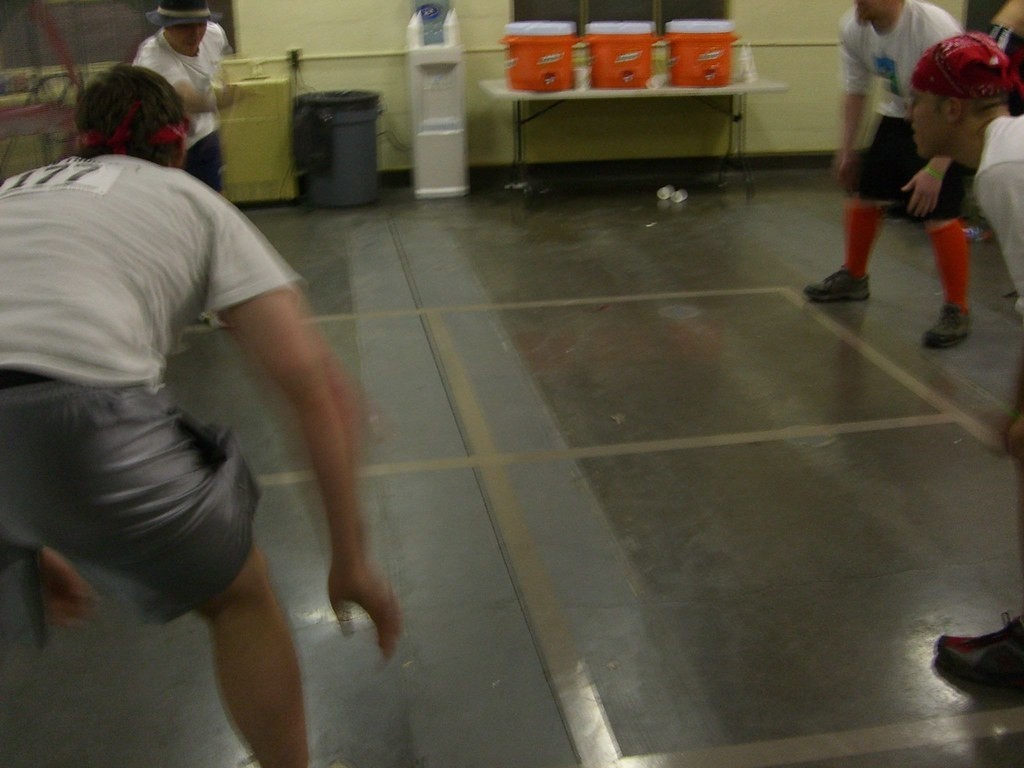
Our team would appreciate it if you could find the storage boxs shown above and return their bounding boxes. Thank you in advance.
[497,20,743,93]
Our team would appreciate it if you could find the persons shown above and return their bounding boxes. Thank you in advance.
[0,65,403,768]
[133,0,239,329]
[805,0,1024,709]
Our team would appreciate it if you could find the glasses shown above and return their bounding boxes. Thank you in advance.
[906,91,933,109]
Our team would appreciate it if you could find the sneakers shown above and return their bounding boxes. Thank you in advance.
[934,618,1024,692]
[804,267,871,302]
[925,302,972,348]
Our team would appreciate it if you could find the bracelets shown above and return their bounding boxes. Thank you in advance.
[924,166,944,179]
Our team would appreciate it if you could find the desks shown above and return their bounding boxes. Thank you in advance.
[482,76,787,195]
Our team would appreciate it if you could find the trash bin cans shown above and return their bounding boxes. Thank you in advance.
[292,89,380,209]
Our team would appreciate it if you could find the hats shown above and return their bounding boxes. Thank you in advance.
[146,0,223,27]
